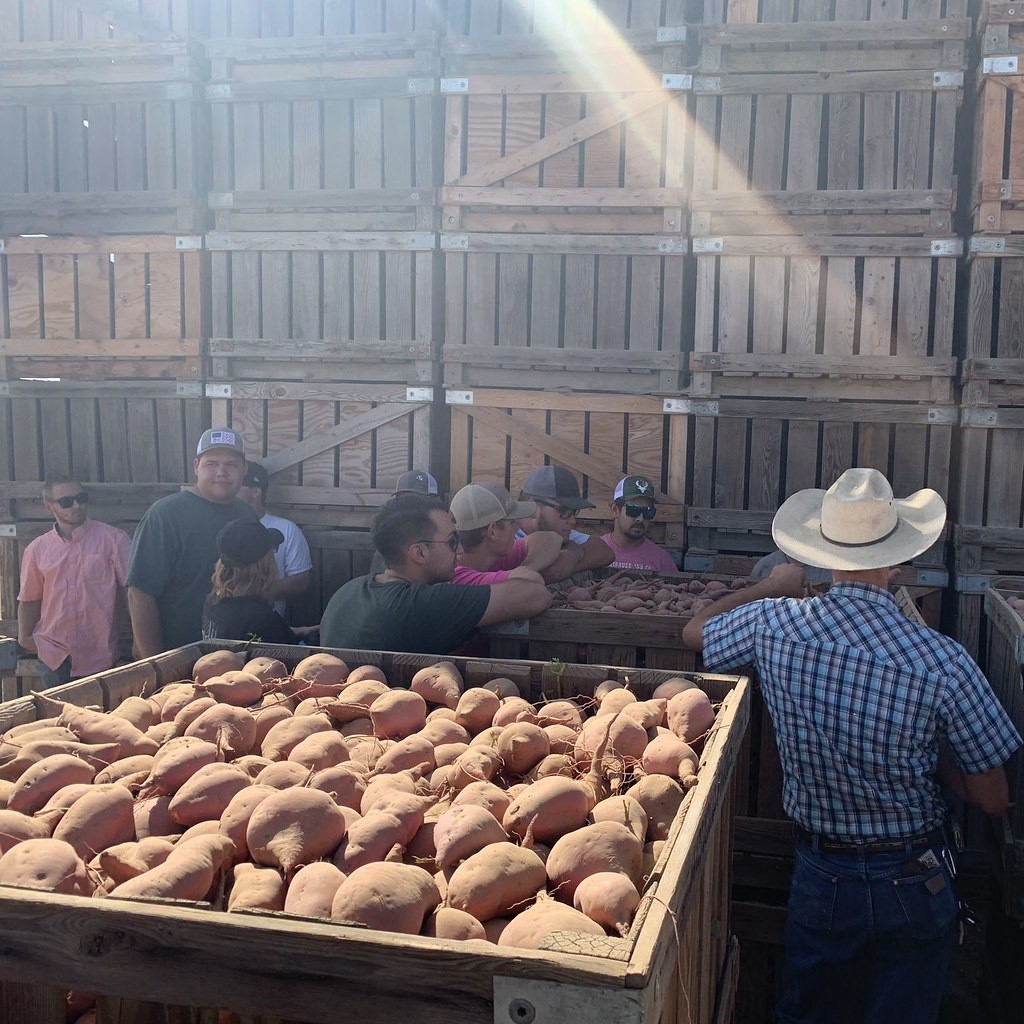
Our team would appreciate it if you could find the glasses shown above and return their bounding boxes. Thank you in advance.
[273,544,279,553]
[538,500,581,519]
[618,502,657,520]
[52,491,89,509]
[415,535,458,553]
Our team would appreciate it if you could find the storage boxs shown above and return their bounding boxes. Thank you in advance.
[0,0,1024,1024]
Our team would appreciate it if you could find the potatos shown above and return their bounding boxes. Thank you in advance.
[1005,595,1024,614]
[549,568,829,615]
[887,568,902,585]
[0,650,713,1024]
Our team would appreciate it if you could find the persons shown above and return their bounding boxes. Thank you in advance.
[319,465,616,654]
[600,475,679,571]
[16,475,132,690]
[682,468,1024,1024]
[751,550,833,582]
[125,427,319,658]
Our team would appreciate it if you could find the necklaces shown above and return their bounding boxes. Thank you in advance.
[784,553,808,567]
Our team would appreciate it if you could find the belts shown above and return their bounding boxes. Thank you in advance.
[797,824,945,856]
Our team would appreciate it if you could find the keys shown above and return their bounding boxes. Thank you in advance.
[955,905,976,945]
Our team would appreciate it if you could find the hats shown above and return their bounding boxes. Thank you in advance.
[613,475,656,502]
[196,427,247,458]
[449,480,538,531]
[242,462,269,488]
[216,517,285,566]
[390,469,438,496]
[521,466,596,509]
[772,468,947,571]
